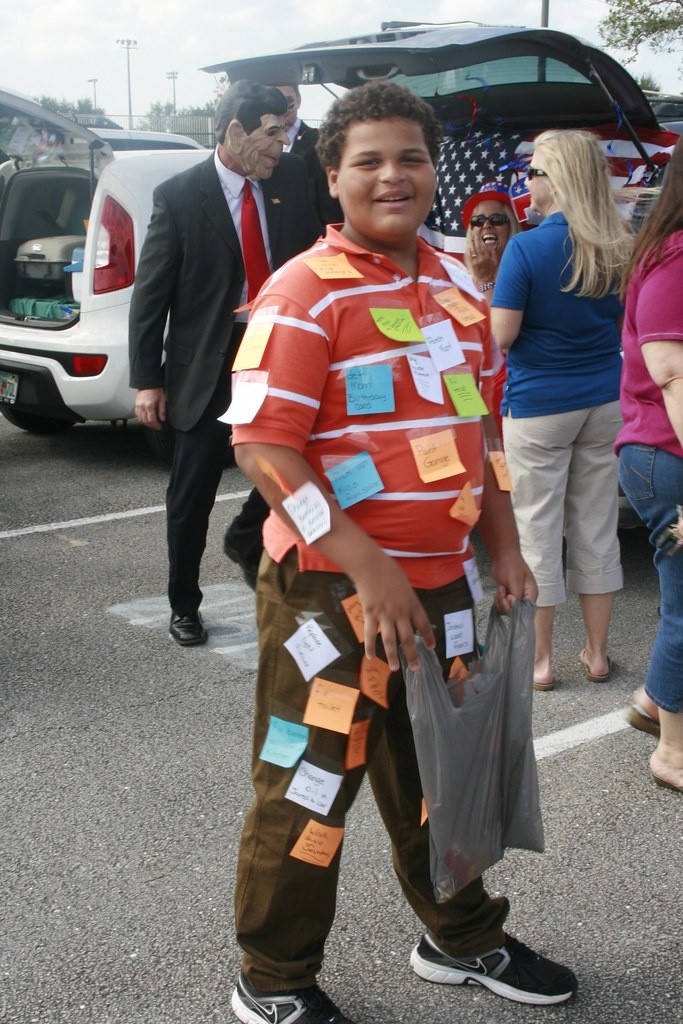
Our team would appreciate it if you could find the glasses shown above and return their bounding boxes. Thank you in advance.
[284,96,296,109]
[526,165,547,179]
[470,213,509,227]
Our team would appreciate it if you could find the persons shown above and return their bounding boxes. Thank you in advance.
[229,84,577,1024]
[463,182,521,443]
[614,134,683,795]
[128,82,321,645]
[275,84,345,226]
[488,128,638,690]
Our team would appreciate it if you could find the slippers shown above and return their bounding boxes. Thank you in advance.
[580,650,612,682]
[530,678,556,691]
[652,774,683,795]
[624,704,660,738]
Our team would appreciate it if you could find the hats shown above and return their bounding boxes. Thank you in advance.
[461,181,510,228]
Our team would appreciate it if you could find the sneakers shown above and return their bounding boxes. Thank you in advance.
[231,973,353,1024]
[408,931,578,1005]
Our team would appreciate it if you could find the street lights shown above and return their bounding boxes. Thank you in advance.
[88,79,98,110]
[116,39,138,129]
[167,71,178,116]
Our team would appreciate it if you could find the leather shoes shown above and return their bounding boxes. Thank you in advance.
[223,538,266,593]
[169,610,206,645]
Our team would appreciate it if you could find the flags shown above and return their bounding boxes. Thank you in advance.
[414,124,679,273]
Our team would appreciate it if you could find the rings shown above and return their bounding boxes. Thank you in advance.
[472,255,477,257]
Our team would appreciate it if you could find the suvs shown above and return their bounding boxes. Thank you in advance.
[198,22,683,529]
[0,89,214,467]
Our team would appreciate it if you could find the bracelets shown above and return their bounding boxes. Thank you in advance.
[478,281,496,291]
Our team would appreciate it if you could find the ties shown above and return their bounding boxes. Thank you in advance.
[242,178,272,307]
